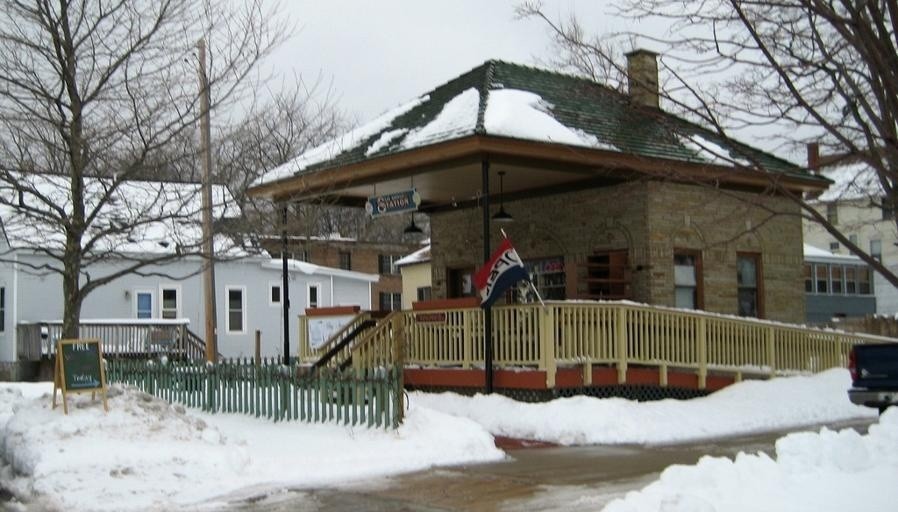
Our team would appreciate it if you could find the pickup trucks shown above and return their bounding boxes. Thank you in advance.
[846,340,898,418]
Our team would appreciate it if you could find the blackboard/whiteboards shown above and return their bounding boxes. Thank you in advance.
[58,337,106,393]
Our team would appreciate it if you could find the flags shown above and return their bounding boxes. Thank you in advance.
[469,239,532,308]
